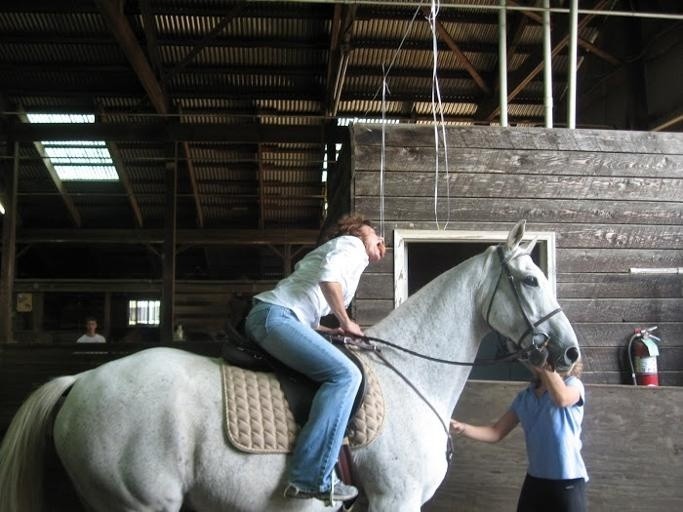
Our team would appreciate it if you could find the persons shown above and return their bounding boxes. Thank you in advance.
[76,316,106,344]
[449,359,590,512]
[244,214,386,501]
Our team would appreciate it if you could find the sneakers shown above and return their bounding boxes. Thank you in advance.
[283,478,359,502]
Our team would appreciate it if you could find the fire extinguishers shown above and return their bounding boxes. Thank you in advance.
[627,326,661,386]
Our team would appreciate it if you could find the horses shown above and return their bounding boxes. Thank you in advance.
[0,220,581,512]
